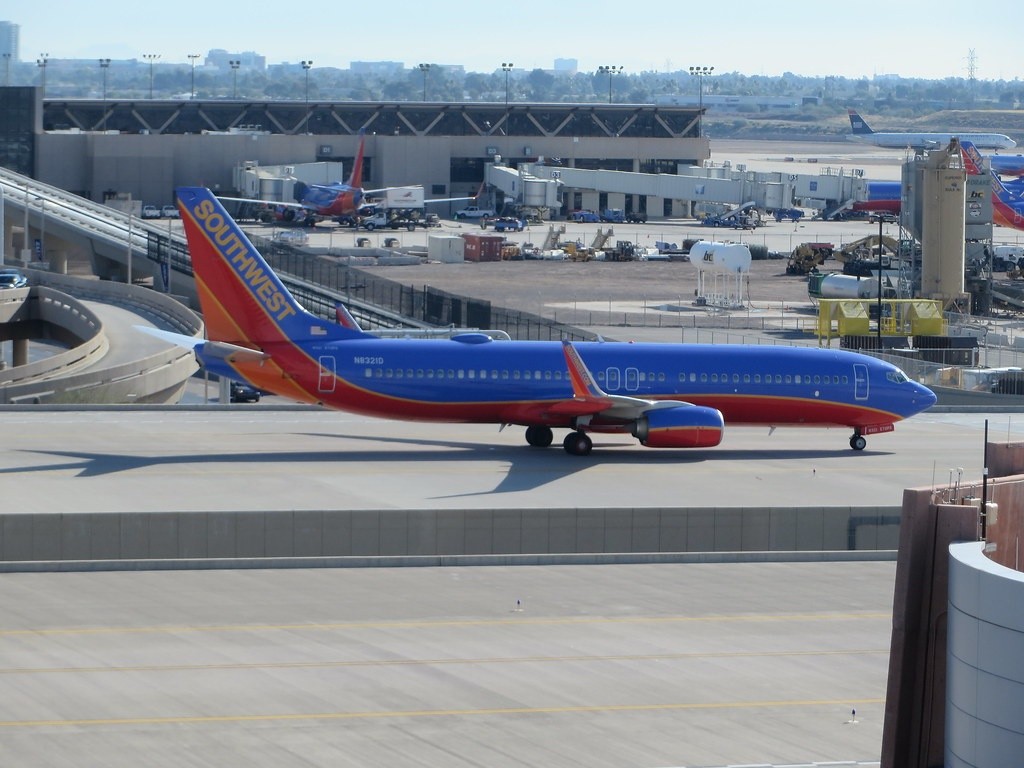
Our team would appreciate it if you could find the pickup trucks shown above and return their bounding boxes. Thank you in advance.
[454,205,494,220]
[161,205,179,219]
[141,205,161,219]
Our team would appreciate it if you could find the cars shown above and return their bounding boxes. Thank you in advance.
[0,267,32,290]
[230,380,260,402]
[571,209,601,223]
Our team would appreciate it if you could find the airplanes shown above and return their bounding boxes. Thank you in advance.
[843,107,1017,155]
[212,127,476,228]
[853,140,1024,233]
[132,181,938,458]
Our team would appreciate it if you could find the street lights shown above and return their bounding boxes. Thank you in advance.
[598,65,625,104]
[99,58,112,134]
[142,54,162,99]
[2,53,12,86]
[229,60,241,100]
[419,63,431,102]
[869,214,897,353]
[36,53,49,98]
[688,66,715,138]
[300,60,314,135]
[502,62,514,136]
[188,55,201,99]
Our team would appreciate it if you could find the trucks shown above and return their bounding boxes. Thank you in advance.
[361,212,425,231]
